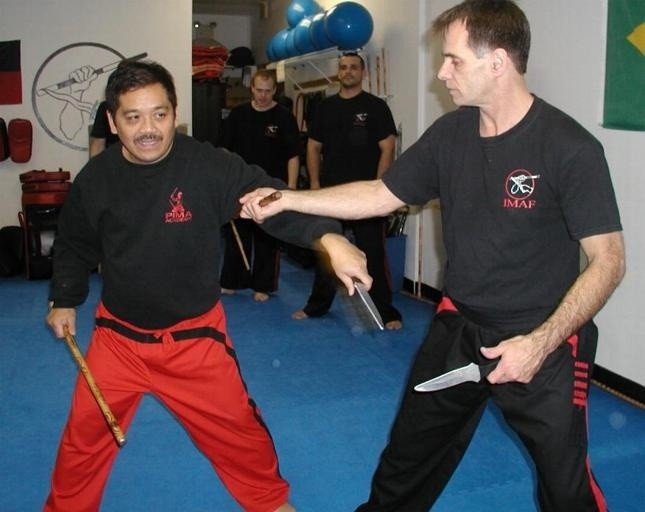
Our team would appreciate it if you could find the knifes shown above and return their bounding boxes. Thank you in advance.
[350,276,386,333]
[412,359,500,393]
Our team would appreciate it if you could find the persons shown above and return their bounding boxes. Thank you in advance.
[236,0,629,512]
[88,58,147,276]
[218,69,305,300]
[290,51,404,330]
[58,65,100,142]
[43,57,375,512]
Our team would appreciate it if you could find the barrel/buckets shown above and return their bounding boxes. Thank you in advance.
[385,232,406,295]
